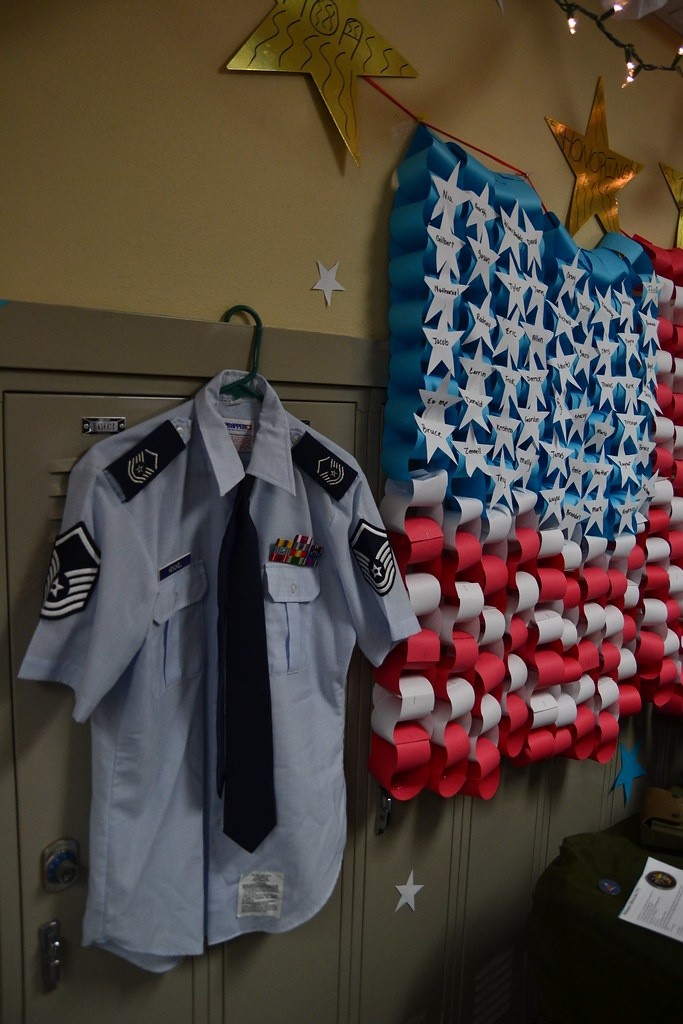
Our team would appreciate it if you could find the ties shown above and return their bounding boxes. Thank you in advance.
[219,473,279,855]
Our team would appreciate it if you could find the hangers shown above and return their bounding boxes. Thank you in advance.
[217,302,264,401]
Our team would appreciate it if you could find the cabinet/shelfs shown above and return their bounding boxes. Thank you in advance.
[0,368,652,1023]
